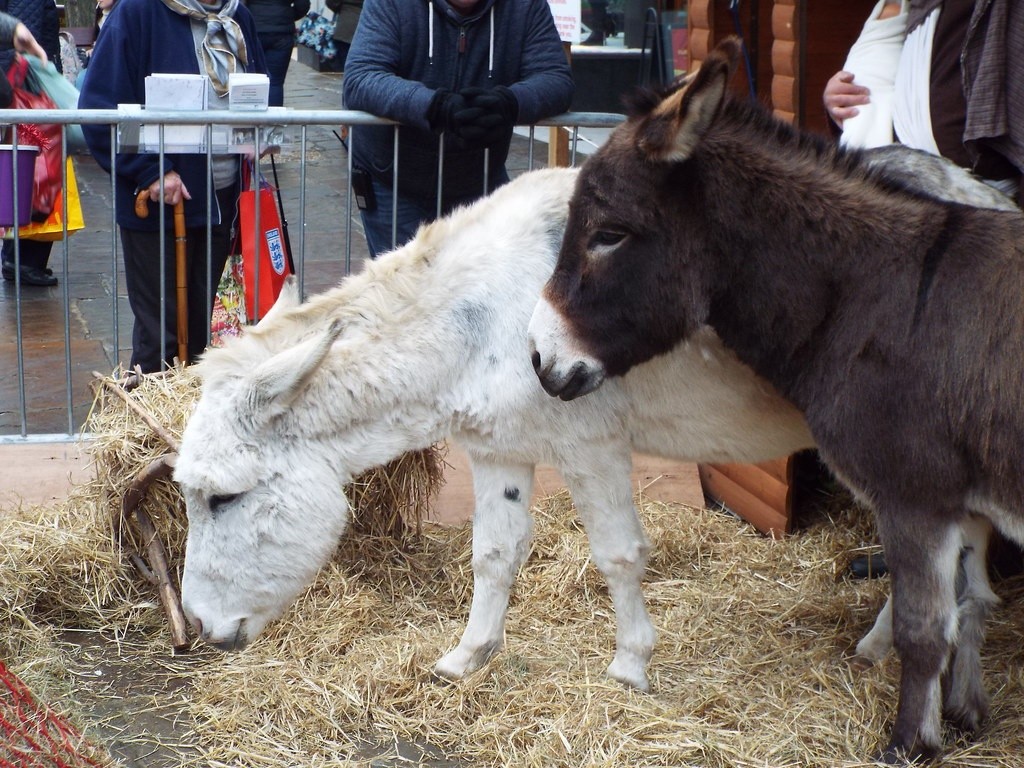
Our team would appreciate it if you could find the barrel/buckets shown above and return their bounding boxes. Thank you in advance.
[0,143,40,228]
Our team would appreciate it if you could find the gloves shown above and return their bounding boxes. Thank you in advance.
[427,82,517,145]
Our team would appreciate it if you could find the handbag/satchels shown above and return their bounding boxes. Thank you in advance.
[239,158,288,325]
[1,51,96,242]
[211,226,246,347]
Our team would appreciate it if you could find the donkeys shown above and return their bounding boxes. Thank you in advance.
[529,57,1024,763]
[175,142,1023,692]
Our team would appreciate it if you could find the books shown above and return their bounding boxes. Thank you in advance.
[145,73,269,146]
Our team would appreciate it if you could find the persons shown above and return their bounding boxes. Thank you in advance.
[326,0,365,66]
[77,0,285,386]
[0,0,62,284]
[244,0,311,107]
[345,0,577,258]
[822,0,1024,576]
[86,0,118,58]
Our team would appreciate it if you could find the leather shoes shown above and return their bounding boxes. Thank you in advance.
[2,258,58,286]
